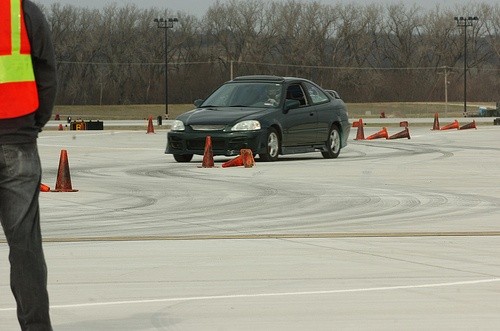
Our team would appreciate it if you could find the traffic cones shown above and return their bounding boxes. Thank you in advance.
[432,113,441,131]
[365,127,389,141]
[353,118,366,140]
[145,114,157,135]
[352,121,359,127]
[388,127,411,140]
[221,149,256,168]
[55,113,61,120]
[400,121,409,128]
[39,183,51,193]
[197,136,219,169]
[58,124,63,130]
[459,120,477,130]
[50,150,80,193]
[440,120,460,131]
[380,112,386,118]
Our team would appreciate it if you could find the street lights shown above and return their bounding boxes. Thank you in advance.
[153,17,179,119]
[453,16,480,113]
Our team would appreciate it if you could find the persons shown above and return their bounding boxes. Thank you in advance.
[264,86,280,103]
[0,0,56,331]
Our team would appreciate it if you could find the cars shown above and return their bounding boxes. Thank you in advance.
[163,75,352,163]
